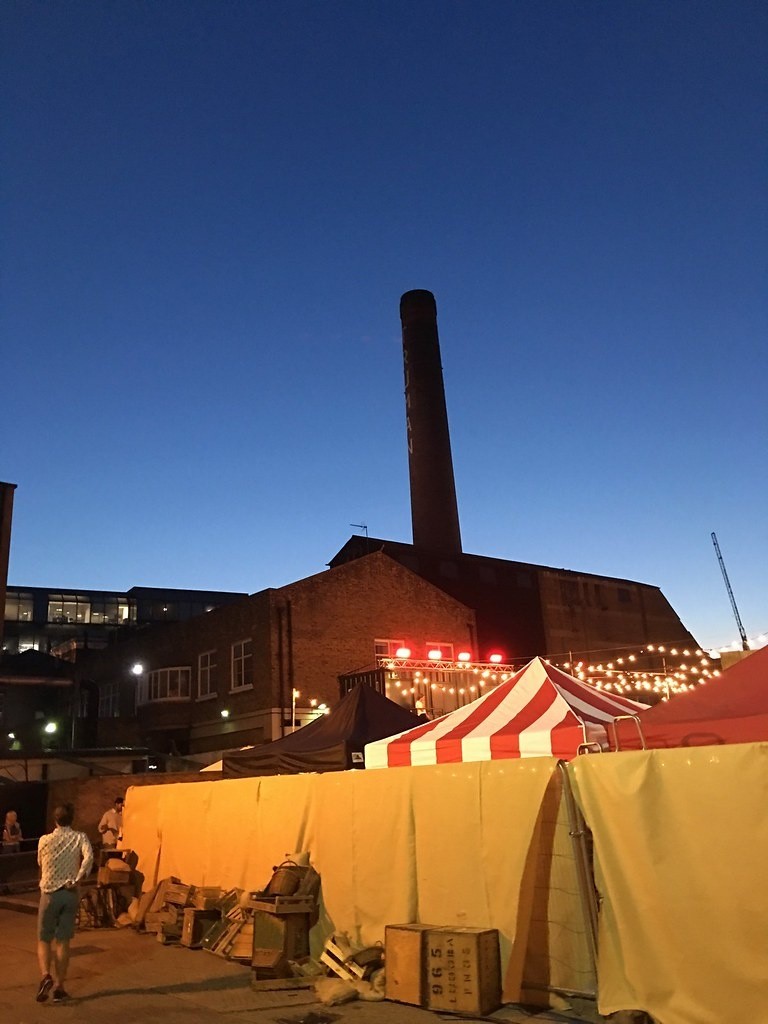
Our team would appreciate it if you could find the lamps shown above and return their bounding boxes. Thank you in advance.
[490,654,502,662]
[458,652,470,661]
[427,650,442,659]
[221,701,229,717]
[396,648,410,659]
[8,730,23,751]
[318,704,326,709]
[308,698,317,706]
[163,604,167,611]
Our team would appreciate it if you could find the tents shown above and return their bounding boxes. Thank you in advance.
[362,657,653,770]
[606,645,768,751]
[221,679,431,778]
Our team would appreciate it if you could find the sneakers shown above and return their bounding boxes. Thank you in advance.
[36,974,54,1003]
[52,990,69,1001]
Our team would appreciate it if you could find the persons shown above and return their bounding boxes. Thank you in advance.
[36,802,93,1002]
[4,811,24,842]
[97,797,124,849]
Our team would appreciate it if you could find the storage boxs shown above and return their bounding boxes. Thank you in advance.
[385,923,440,1006]
[426,926,503,1018]
[99,867,130,884]
[144,875,244,949]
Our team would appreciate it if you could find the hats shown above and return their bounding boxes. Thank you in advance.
[114,797,124,803]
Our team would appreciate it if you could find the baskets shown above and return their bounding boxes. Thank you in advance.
[343,940,385,967]
[269,860,300,896]
